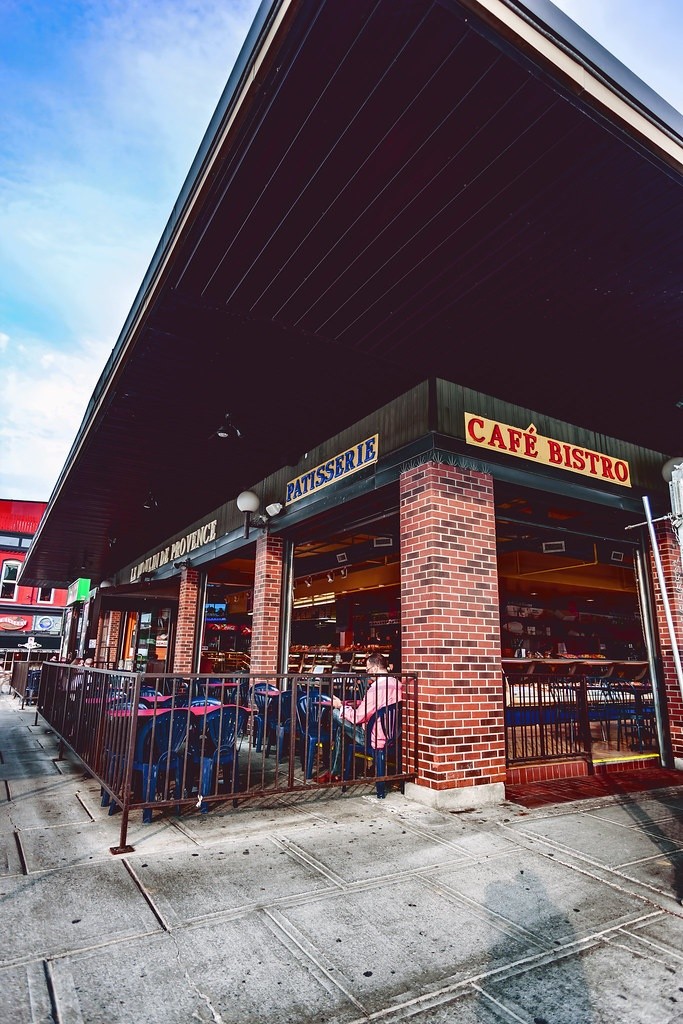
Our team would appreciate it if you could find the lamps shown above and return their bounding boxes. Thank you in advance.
[236,491,269,540]
[265,502,286,517]
[661,455,683,523]
[97,580,120,595]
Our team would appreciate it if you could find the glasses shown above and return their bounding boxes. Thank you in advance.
[366,666,373,673]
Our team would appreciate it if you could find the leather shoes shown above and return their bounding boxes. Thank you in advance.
[314,771,341,783]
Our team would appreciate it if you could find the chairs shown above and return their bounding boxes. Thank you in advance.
[25,668,43,703]
[548,676,659,754]
[68,672,405,825]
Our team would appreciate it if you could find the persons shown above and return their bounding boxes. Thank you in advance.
[314,653,402,784]
[84,657,93,667]
